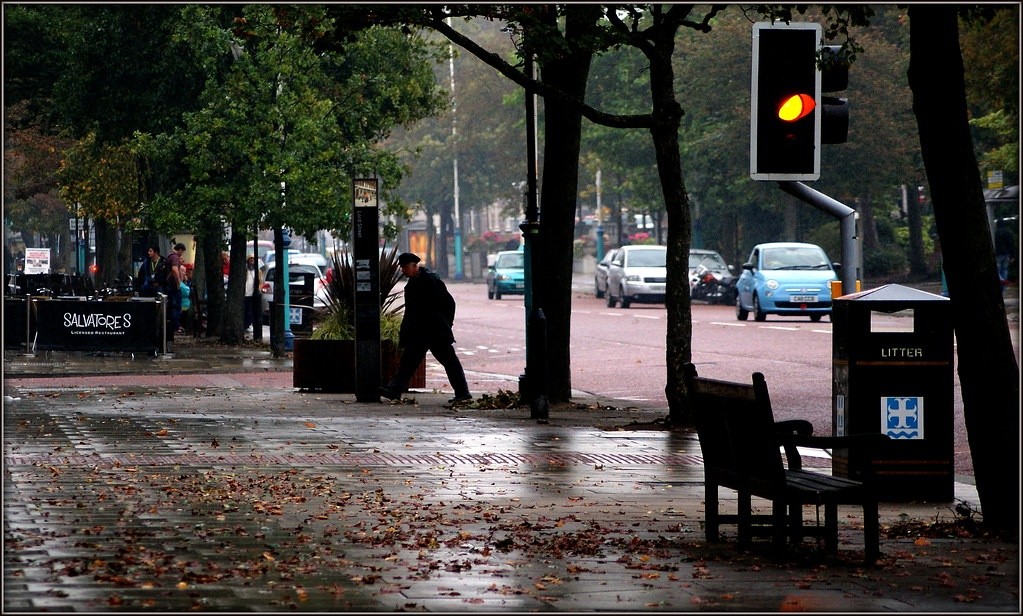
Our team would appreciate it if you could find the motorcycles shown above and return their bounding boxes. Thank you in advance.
[689,254,738,305]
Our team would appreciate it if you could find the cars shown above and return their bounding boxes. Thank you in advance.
[245,240,341,324]
[688,249,734,298]
[595,244,667,308]
[736,242,841,323]
[488,250,523,300]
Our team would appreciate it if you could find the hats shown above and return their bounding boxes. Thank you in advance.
[184,264,192,273]
[398,253,421,267]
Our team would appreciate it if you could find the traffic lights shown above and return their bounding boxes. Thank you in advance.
[821,45,850,145]
[750,21,820,182]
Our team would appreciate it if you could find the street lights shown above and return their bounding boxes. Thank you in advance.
[67,183,81,277]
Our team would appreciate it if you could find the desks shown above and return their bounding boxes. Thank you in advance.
[3,296,159,355]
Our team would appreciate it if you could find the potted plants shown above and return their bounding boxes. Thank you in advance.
[292,242,426,391]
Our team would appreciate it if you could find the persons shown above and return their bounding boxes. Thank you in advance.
[138,243,264,331]
[994,218,1016,292]
[380,253,473,404]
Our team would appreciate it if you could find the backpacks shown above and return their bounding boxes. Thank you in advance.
[153,254,181,284]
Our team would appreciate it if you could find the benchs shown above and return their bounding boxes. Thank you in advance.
[682,363,880,570]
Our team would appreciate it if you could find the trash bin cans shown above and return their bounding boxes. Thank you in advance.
[834,283,954,504]
[288,264,315,332]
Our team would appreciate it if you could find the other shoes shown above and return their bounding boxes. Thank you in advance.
[378,380,404,401]
[167,341,177,349]
[251,338,263,345]
[448,387,472,403]
